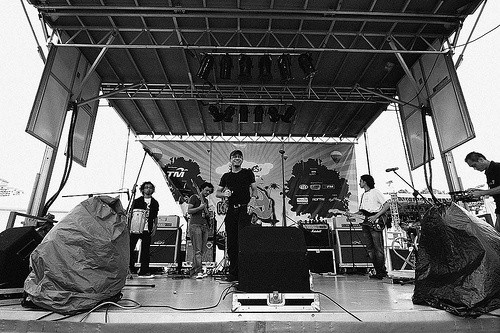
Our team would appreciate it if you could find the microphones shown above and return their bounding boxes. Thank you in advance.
[143,146,153,157]
[385,168,399,172]
[127,188,130,201]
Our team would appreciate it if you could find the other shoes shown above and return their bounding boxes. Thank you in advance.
[369,274,387,279]
[194,273,208,277]
[195,272,203,279]
[138,272,155,278]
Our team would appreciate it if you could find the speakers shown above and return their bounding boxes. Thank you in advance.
[185,219,217,262]
[149,227,183,263]
[0,227,45,289]
[236,227,375,294]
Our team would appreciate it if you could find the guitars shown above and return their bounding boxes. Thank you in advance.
[191,179,217,230]
[328,209,385,232]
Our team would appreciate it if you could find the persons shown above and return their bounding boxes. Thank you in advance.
[344,174,389,279]
[465,152,500,233]
[187,182,214,279]
[216,150,258,281]
[37,213,56,237]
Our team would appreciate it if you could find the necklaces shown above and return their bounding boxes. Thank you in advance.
[128,182,158,277]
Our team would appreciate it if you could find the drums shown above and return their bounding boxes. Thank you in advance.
[126,209,147,234]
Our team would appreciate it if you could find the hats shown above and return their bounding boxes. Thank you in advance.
[230,150,242,156]
